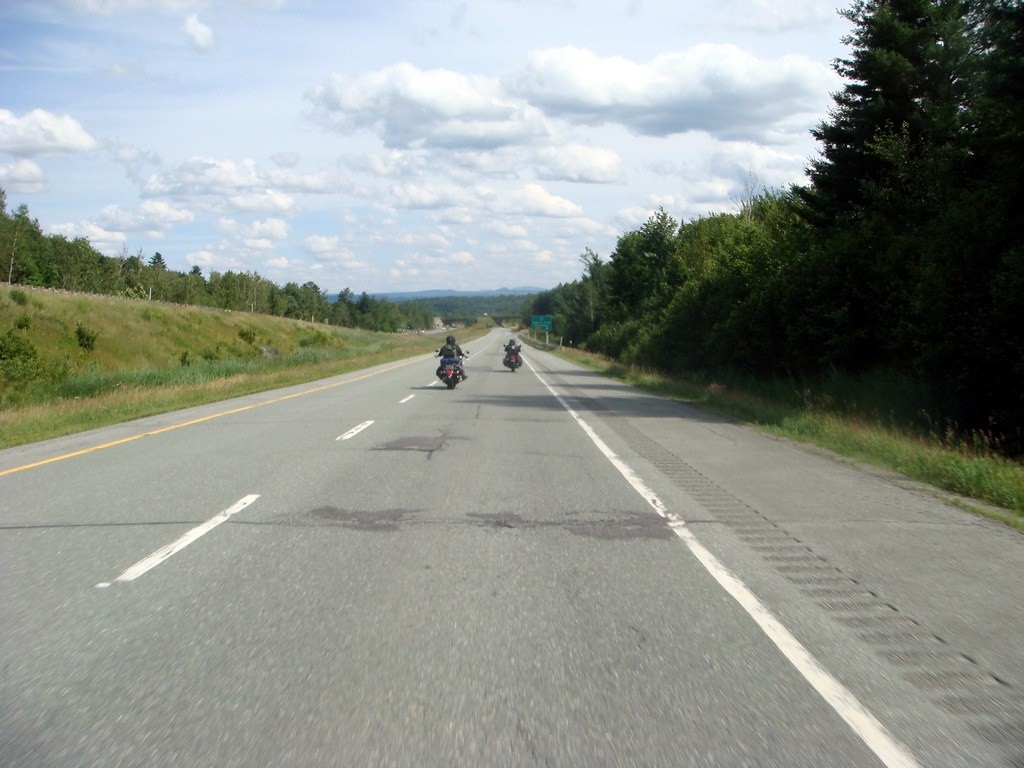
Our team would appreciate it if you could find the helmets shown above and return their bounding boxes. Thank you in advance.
[446,336,455,344]
[509,339,515,344]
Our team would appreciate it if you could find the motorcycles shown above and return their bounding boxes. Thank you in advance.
[435,349,470,388]
[503,344,524,372]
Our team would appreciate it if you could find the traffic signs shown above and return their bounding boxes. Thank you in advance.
[531,315,553,332]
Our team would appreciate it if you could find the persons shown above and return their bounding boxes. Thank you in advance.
[435,335,467,381]
[504,339,522,365]
